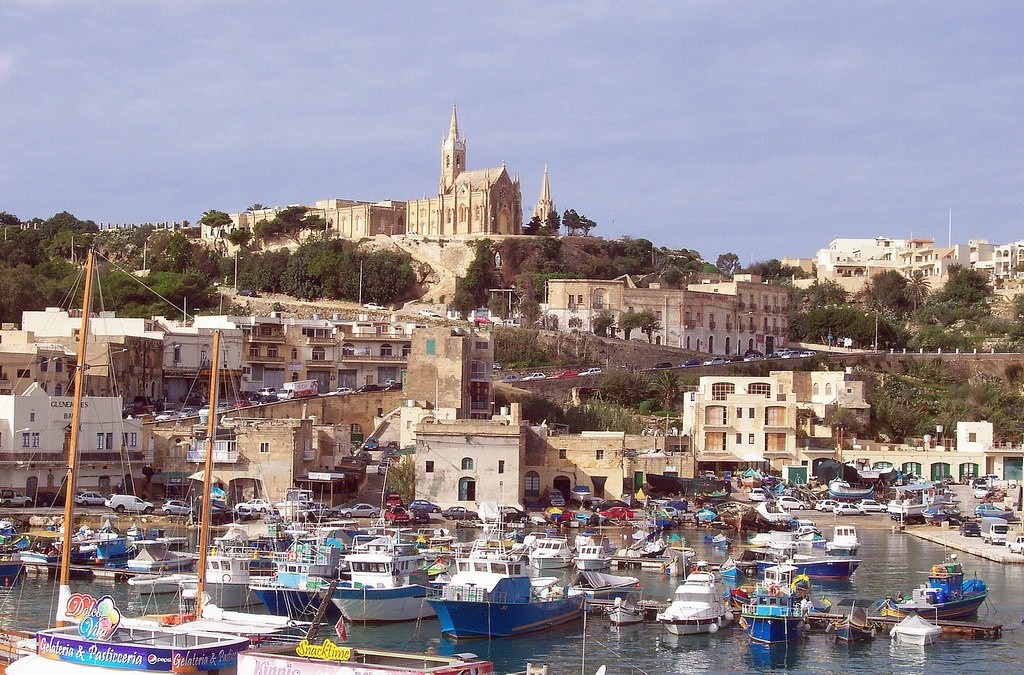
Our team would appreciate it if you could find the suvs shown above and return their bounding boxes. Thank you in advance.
[104,493,156,514]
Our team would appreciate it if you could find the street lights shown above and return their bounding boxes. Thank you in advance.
[605,321,619,371]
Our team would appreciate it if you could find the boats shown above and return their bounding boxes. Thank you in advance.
[0,247,992,675]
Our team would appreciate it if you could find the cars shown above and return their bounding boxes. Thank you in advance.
[31,490,66,507]
[339,502,381,519]
[74,491,106,507]
[311,503,340,517]
[235,498,271,513]
[161,499,195,516]
[132,285,819,417]
[360,435,1024,558]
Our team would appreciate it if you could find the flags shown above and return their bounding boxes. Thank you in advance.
[335,615,347,643]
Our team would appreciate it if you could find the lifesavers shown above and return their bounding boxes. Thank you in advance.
[222,574,232,582]
[769,585,780,597]
[287,550,298,562]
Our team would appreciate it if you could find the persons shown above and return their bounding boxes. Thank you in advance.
[842,334,852,352]
[33,540,42,552]
[44,544,58,554]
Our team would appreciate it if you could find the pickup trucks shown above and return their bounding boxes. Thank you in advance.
[0,489,33,508]
